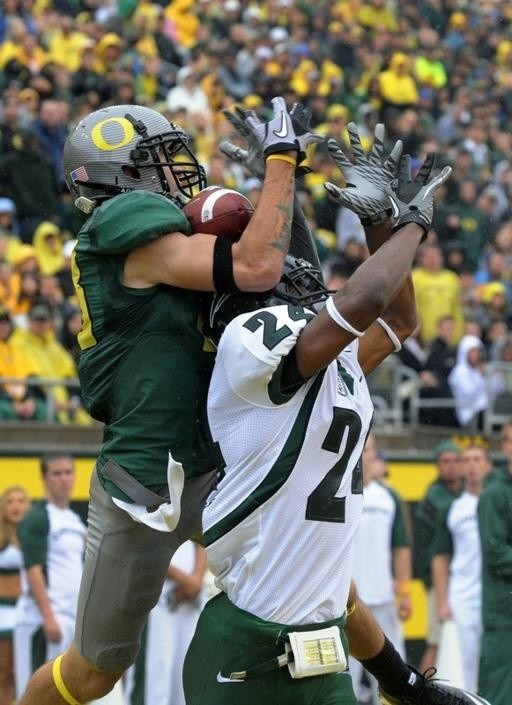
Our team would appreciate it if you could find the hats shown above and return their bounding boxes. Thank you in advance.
[0,199,16,213]
[29,305,49,318]
[436,442,460,457]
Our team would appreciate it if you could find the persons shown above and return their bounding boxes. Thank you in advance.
[1,3,511,704]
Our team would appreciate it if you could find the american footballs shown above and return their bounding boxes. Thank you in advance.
[184,185,254,237]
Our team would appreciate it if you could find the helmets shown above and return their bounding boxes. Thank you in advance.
[203,254,338,353]
[62,103,208,220]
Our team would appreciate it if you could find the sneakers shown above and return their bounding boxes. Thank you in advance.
[378,664,488,704]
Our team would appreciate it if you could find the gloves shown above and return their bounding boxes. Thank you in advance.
[218,97,326,179]
[384,150,453,244]
[323,124,403,227]
